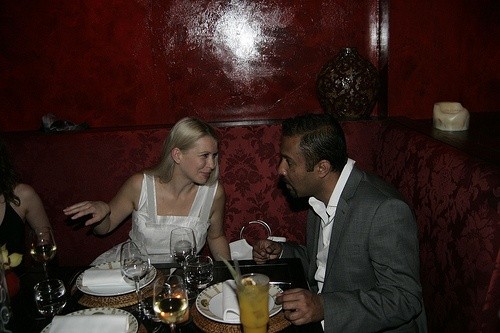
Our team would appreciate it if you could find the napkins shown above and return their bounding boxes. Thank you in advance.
[223,279,242,322]
[50,315,131,333]
[82,270,130,288]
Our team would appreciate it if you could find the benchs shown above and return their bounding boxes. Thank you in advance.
[0,114,500,333]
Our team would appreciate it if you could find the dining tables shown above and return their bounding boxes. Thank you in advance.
[13,258,325,333]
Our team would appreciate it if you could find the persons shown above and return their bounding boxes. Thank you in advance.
[64,117,232,264]
[253,113,427,333]
[0,151,53,254]
